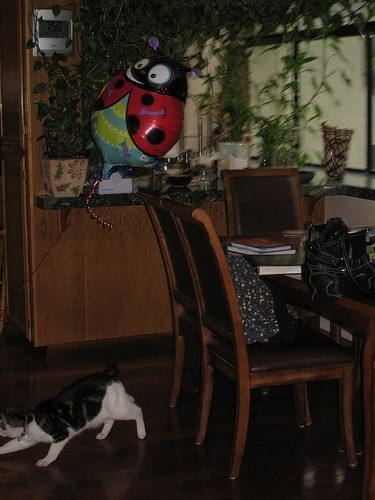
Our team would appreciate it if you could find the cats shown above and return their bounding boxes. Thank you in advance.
[1,363,147,467]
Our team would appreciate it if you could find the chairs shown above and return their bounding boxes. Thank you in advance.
[136,189,357,480]
[221,166,304,232]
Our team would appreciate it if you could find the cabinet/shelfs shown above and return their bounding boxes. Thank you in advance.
[0,0,223,350]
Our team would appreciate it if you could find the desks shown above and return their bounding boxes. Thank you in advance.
[260,276,375,491]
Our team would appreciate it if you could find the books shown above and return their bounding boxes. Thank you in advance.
[231,236,292,252]
[226,245,296,255]
[241,254,302,276]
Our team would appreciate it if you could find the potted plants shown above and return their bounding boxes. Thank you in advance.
[201,37,251,169]
[31,43,91,197]
[259,47,310,166]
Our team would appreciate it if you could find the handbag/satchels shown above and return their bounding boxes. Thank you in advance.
[301,217,375,303]
[224,254,279,344]
